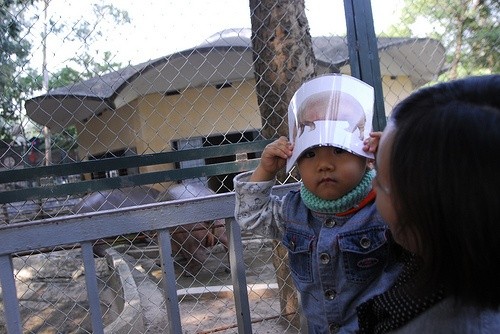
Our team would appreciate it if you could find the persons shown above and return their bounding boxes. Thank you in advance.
[369,70,500,333]
[232,131,403,334]
